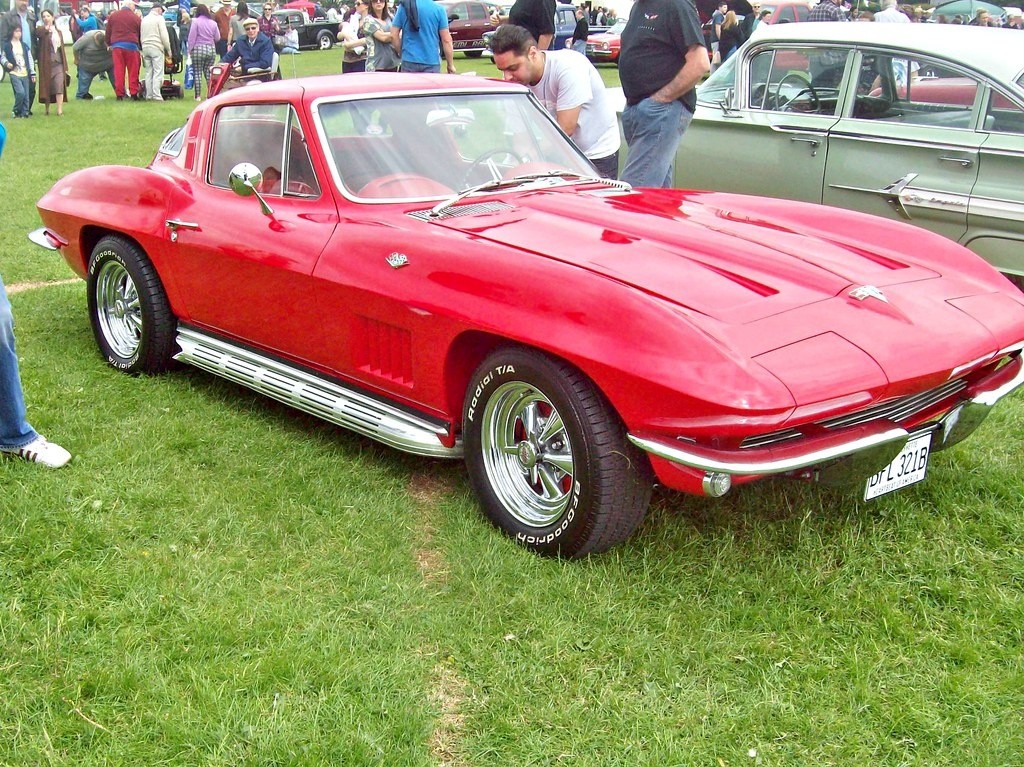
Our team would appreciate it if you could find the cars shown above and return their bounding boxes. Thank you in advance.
[545,2,580,54]
[867,70,1022,108]
[564,18,629,66]
[756,1,818,71]
[436,0,512,60]
[604,20,1024,284]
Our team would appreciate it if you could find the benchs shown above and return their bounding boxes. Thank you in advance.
[876,111,996,131]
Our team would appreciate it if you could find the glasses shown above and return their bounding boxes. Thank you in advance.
[353,1,366,6]
[752,6,760,9]
[371,0,386,4]
[264,8,272,10]
[246,26,256,31]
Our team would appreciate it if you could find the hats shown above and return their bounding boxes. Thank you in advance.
[242,18,258,27]
[220,0,232,5]
[152,2,165,13]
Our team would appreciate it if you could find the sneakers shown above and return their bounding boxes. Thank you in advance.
[0,434,71,468]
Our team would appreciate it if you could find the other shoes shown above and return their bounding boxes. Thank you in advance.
[194,95,201,101]
[22,113,29,119]
[100,74,107,80]
[12,110,21,119]
[130,93,138,100]
[116,95,123,100]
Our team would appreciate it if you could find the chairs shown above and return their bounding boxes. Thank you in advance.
[852,97,891,120]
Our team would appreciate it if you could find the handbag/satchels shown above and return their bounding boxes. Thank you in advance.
[711,49,721,66]
[275,34,287,48]
[183,53,195,90]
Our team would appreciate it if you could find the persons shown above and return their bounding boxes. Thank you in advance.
[617,0,711,191]
[0,122,72,468]
[489,25,621,182]
[0,0,1024,118]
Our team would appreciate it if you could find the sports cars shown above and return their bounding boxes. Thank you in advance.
[27,69,1024,562]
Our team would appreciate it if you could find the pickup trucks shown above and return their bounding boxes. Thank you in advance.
[269,8,341,52]
